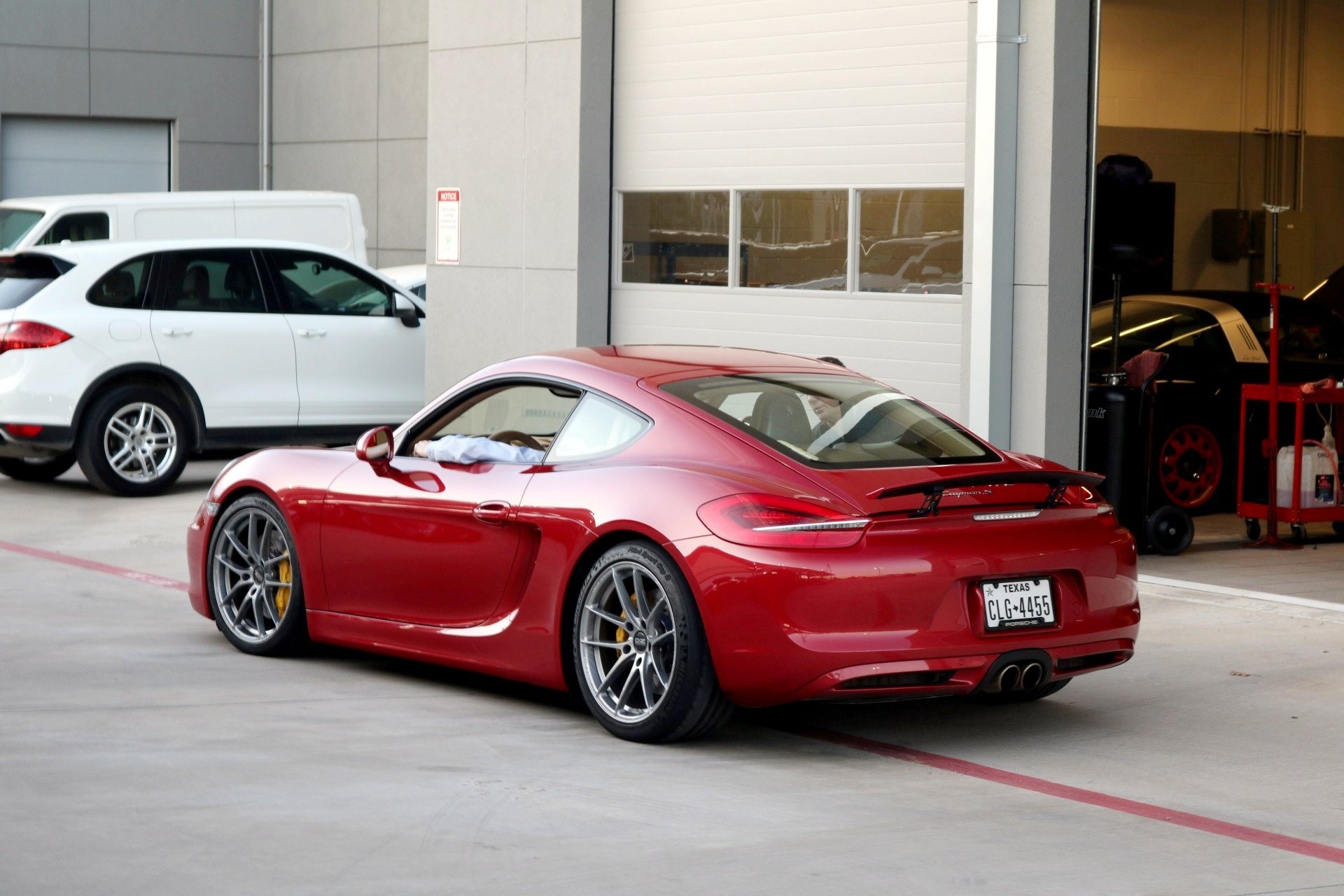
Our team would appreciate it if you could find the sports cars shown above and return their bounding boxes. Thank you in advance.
[184,347,1146,750]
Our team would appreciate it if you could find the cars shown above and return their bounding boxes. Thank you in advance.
[380,263,427,300]
[1294,268,1344,313]
[1081,289,1344,518]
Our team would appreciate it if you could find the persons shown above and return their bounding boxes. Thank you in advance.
[413,434,546,463]
[811,357,847,442]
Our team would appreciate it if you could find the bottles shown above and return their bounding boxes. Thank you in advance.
[1321,425,1336,449]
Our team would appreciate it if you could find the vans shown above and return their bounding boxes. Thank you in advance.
[0,190,370,259]
[858,230,963,290]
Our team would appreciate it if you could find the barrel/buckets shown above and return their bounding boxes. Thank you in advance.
[1276,444,1338,507]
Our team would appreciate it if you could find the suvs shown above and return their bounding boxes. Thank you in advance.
[0,238,431,500]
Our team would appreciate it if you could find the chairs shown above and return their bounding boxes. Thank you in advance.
[215,264,258,311]
[753,391,810,447]
[185,264,210,303]
[107,272,138,304]
